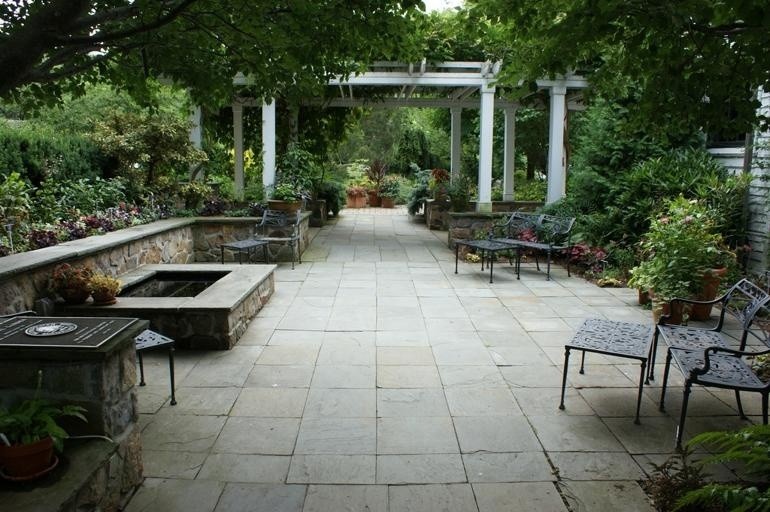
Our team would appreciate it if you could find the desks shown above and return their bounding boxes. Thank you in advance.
[221,240,269,264]
[559,319,655,425]
[134,329,177,405]
[455,240,521,283]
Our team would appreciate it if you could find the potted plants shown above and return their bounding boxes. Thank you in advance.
[268,177,311,214]
[0,369,88,488]
[365,158,400,208]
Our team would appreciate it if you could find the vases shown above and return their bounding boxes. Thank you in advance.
[347,195,366,208]
[639,266,727,324]
[92,291,117,306]
[60,289,91,305]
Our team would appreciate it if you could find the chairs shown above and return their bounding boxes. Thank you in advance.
[255,209,301,270]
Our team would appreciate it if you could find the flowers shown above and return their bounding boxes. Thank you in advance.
[627,192,737,298]
[47,262,97,292]
[87,273,122,297]
[346,185,368,199]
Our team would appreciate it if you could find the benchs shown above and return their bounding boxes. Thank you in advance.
[486,211,577,281]
[650,277,770,452]
